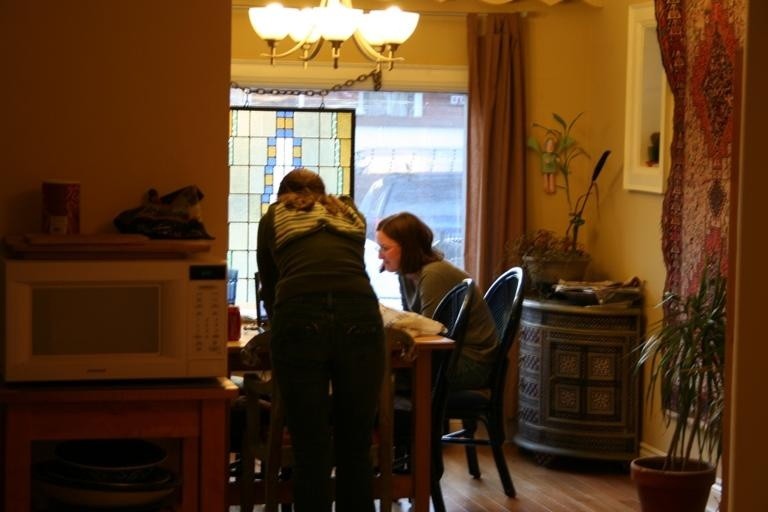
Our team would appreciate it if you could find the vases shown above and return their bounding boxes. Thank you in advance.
[522,253,589,286]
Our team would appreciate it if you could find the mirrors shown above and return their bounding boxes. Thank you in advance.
[618,3,673,197]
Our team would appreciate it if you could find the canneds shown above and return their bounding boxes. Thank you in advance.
[227,305,241,341]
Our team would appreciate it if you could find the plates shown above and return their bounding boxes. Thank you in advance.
[33,446,179,506]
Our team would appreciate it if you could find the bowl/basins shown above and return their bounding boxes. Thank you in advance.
[550,281,640,306]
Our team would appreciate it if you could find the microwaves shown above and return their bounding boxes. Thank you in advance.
[4,260,227,382]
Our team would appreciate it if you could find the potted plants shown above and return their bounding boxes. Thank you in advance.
[616,251,728,511]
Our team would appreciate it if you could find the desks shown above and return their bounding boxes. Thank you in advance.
[514,298,645,473]
[228,302,435,512]
[0,375,239,511]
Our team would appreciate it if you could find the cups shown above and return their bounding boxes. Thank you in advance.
[227,269,241,341]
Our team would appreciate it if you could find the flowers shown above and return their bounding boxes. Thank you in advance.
[645,132,660,168]
[525,106,617,257]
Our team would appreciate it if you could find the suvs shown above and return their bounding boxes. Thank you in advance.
[355,170,464,267]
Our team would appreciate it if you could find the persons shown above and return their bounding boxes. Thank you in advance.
[257,170,385,511]
[377,213,499,474]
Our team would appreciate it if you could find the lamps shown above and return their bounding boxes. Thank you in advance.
[243,0,422,69]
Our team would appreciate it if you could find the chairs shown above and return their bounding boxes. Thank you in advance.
[438,266,532,501]
[253,271,271,325]
[265,328,412,512]
